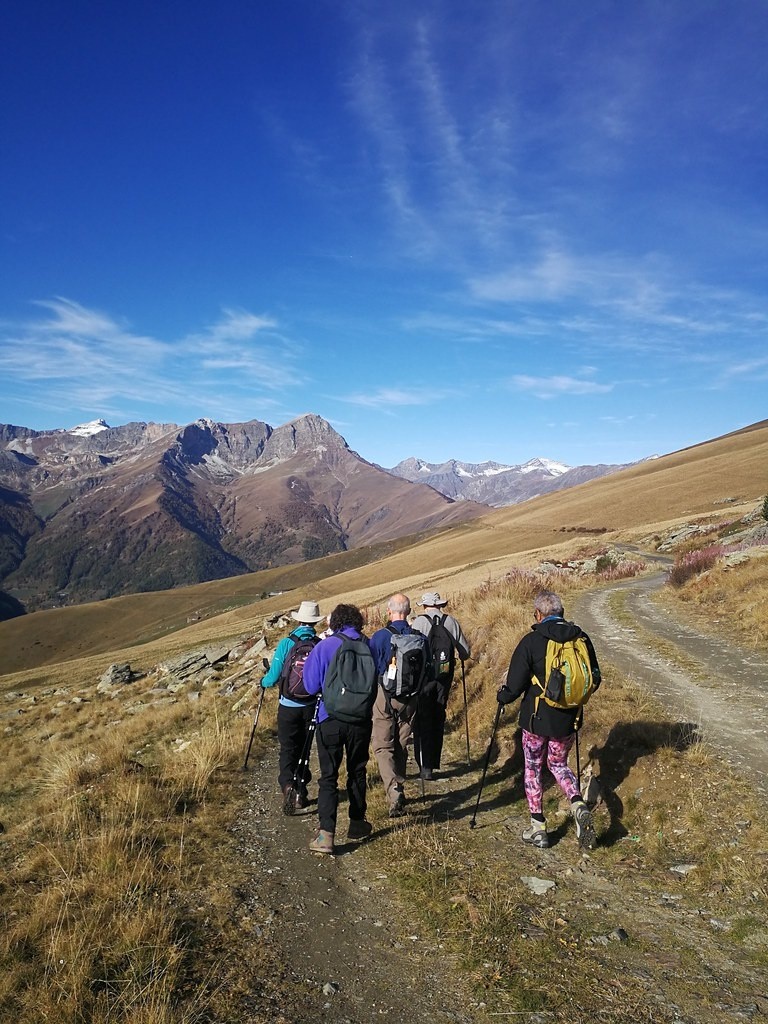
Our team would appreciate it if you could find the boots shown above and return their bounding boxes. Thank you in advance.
[522,818,549,848]
[570,801,598,849]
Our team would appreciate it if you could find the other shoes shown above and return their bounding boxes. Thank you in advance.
[388,784,405,818]
[295,794,310,809]
[309,830,334,853]
[419,766,432,779]
[283,784,296,815]
[347,817,372,837]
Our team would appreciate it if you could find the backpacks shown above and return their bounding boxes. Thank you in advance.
[383,625,428,701]
[531,630,593,709]
[323,634,376,721]
[419,614,455,680]
[279,634,323,703]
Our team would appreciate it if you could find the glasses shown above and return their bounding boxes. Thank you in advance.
[534,610,541,620]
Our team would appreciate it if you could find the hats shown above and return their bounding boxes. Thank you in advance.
[291,601,326,623]
[416,592,449,608]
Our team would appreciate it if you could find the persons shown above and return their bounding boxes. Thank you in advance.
[411,593,471,780]
[319,615,334,639]
[260,601,327,817]
[372,594,433,818]
[303,605,378,852]
[496,593,594,849]
